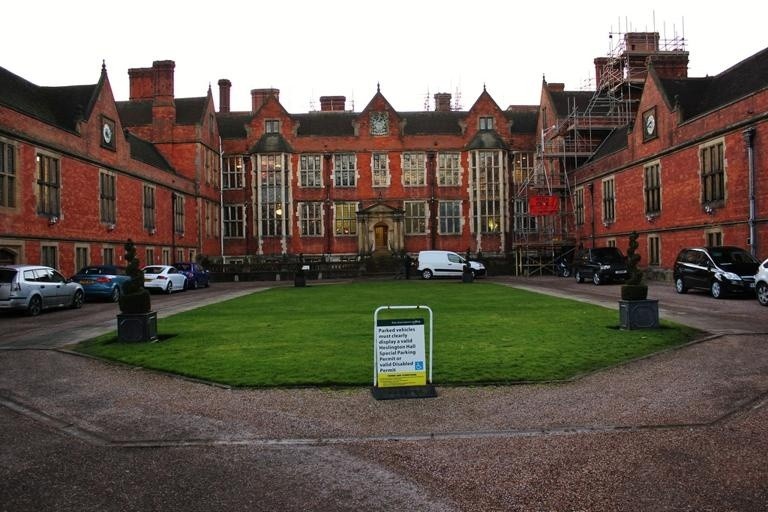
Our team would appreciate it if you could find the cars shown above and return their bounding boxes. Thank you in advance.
[754,258,768,306]
[673,247,762,299]
[70,262,210,302]
[574,247,630,285]
[0,265,85,316]
[416,250,486,280]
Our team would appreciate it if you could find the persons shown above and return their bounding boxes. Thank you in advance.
[404,251,414,280]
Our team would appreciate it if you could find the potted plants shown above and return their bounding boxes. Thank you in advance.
[295,252,305,286]
[618,231,659,330]
[117,238,159,343]
[462,247,473,283]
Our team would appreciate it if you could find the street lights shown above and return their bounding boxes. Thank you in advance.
[541,124,560,152]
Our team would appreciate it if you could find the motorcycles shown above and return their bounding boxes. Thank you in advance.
[555,257,570,278]
